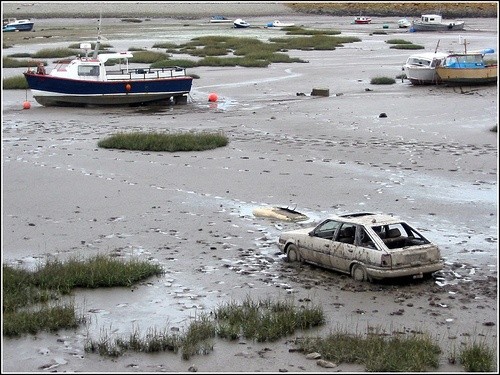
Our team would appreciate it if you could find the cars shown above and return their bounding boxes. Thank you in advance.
[275,212,443,283]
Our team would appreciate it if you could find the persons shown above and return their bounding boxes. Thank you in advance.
[87,66,98,75]
[37,66,45,75]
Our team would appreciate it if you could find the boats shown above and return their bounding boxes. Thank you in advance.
[403,37,497,85]
[3,17,35,32]
[210,15,233,22]
[354,17,372,24]
[234,18,250,28]
[21,12,200,107]
[399,14,465,31]
[272,19,295,27]
